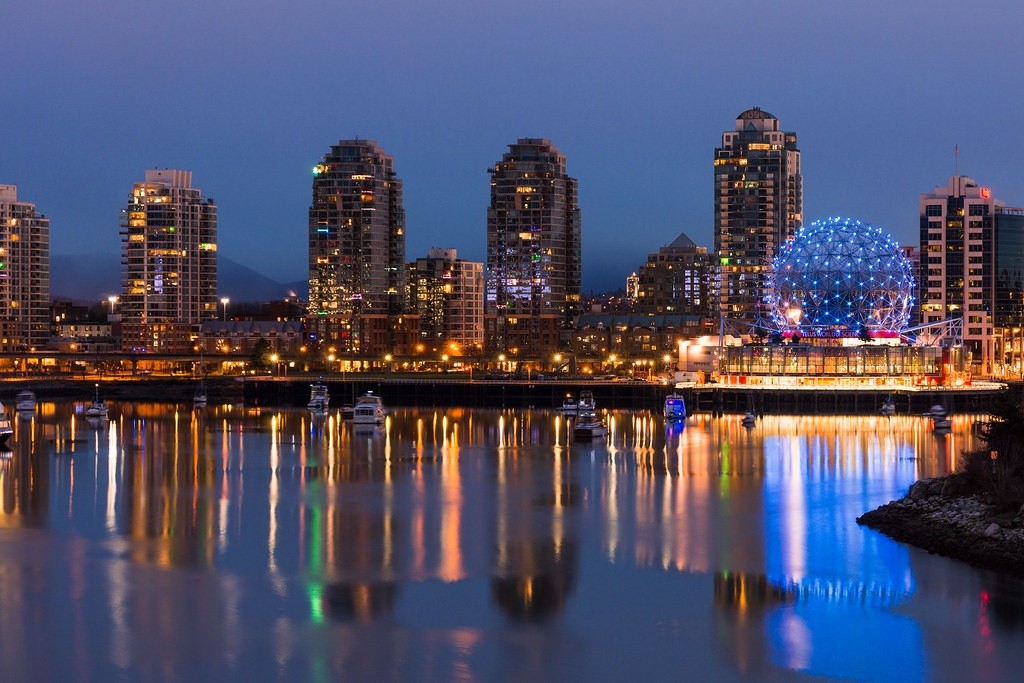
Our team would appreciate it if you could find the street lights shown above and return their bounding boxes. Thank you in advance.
[554,354,561,375]
[664,355,671,379]
[499,355,505,380]
[108,296,118,314]
[610,356,617,373]
[221,298,230,321]
[636,361,641,373]
[642,361,648,372]
[650,361,653,381]
[632,362,636,380]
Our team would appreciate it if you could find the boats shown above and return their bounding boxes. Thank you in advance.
[663,391,686,419]
[556,389,608,438]
[742,412,755,424]
[14,389,38,411]
[926,404,951,429]
[306,384,330,410]
[881,395,897,411]
[340,390,389,424]
[84,382,110,415]
[0,401,15,449]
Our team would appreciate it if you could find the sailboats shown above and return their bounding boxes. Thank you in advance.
[192,352,208,402]
